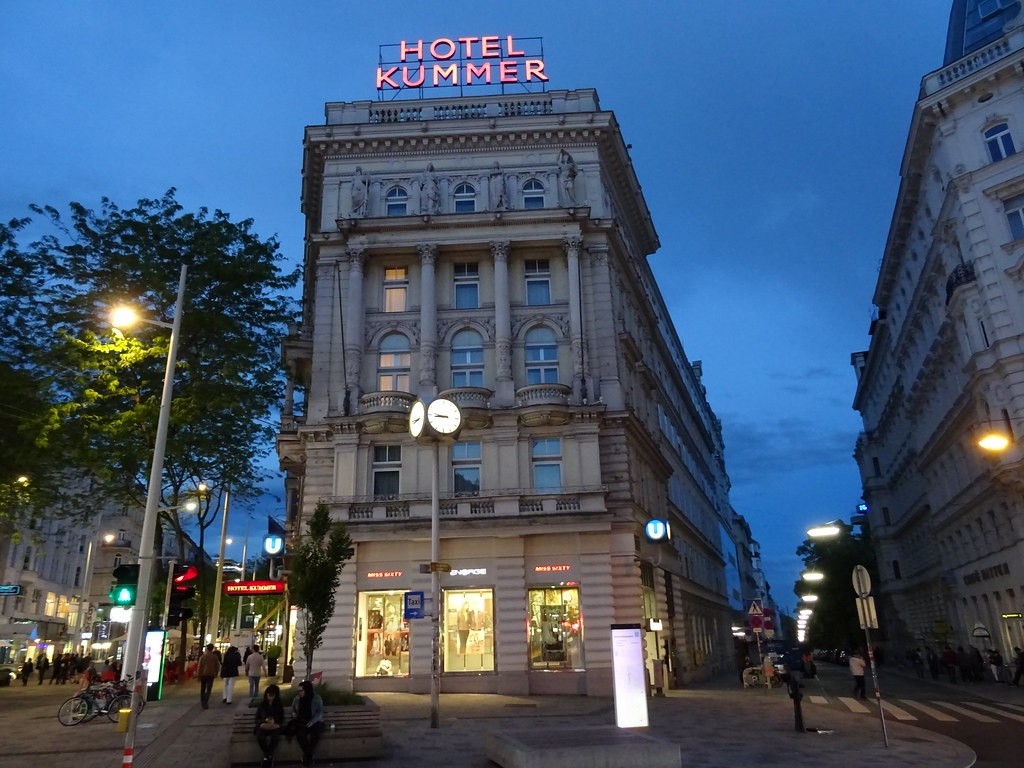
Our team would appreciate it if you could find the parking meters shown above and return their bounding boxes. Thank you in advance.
[762,656,775,689]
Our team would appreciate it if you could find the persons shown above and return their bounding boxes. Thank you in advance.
[196,644,221,709]
[20,651,122,687]
[168,647,253,665]
[291,681,326,768]
[253,685,284,768]
[220,647,242,704]
[848,650,867,700]
[369,604,409,656]
[469,601,486,652]
[913,645,1024,689]
[245,645,268,698]
[457,601,472,654]
[374,657,393,676]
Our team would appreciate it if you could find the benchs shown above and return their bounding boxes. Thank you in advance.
[229,706,383,763]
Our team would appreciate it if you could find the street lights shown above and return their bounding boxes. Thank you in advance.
[109,263,188,694]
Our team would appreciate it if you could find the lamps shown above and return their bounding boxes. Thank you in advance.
[644,518,671,543]
[261,534,285,559]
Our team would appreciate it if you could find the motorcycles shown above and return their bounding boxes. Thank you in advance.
[739,656,783,689]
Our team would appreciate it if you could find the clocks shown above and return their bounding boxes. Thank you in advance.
[408,399,428,439]
[427,399,463,436]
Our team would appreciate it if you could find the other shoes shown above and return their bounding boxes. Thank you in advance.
[223,699,226,703]
[203,704,208,708]
[853,693,858,696]
[23,681,65,685]
[227,702,232,704]
[262,756,273,768]
[860,696,867,700]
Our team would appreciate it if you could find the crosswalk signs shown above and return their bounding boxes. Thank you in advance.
[745,598,763,616]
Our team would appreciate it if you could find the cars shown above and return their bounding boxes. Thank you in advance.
[809,648,855,666]
[764,654,789,676]
[786,648,804,672]
[0,667,16,686]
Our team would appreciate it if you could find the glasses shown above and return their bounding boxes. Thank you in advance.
[297,689,304,692]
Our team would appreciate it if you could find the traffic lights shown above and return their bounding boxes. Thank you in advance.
[107,563,140,605]
[163,562,198,631]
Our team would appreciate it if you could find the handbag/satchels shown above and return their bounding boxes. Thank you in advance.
[260,723,280,730]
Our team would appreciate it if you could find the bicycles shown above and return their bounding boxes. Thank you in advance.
[57,671,144,726]
